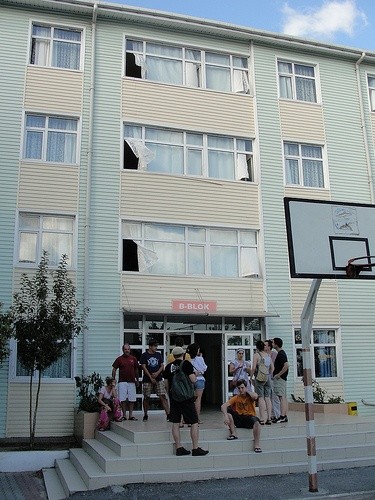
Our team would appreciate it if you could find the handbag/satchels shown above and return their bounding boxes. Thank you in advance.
[256,363,268,383]
[229,381,237,391]
[171,370,194,401]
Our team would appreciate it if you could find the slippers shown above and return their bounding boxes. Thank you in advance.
[227,435,238,440]
[255,447,262,452]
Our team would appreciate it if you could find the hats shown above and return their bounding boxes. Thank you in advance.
[173,348,184,354]
[237,348,244,352]
[149,338,158,343]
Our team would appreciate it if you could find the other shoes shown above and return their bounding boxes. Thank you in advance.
[128,417,135,420]
[277,415,288,423]
[176,447,190,456]
[259,421,264,425]
[121,417,127,421]
[112,418,122,422]
[265,419,271,424]
[143,416,148,421]
[192,447,209,456]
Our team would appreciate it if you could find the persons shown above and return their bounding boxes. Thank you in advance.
[221,379,262,453]
[112,343,139,420]
[140,339,170,421]
[246,338,289,425]
[187,343,208,424]
[167,338,191,426]
[98,379,122,425]
[230,348,252,397]
[163,347,209,456]
